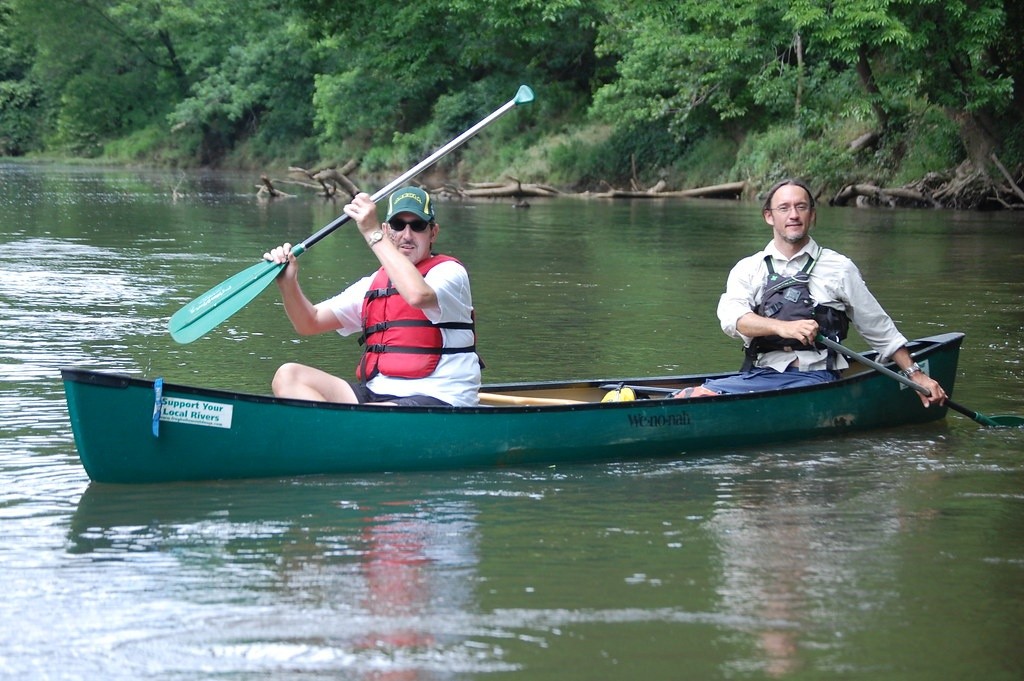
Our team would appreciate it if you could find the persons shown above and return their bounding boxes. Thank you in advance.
[702,180,948,408]
[263,186,482,408]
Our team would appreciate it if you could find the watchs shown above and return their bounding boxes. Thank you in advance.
[368,230,385,248]
[904,362,923,380]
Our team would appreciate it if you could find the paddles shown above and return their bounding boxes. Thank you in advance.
[813,333,1024,425]
[164,86,534,344]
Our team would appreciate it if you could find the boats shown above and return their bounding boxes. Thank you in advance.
[57,330,964,484]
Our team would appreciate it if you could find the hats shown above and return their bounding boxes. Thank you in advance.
[385,186,435,223]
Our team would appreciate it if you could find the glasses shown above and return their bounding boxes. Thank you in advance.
[388,218,429,232]
[766,203,811,213]
